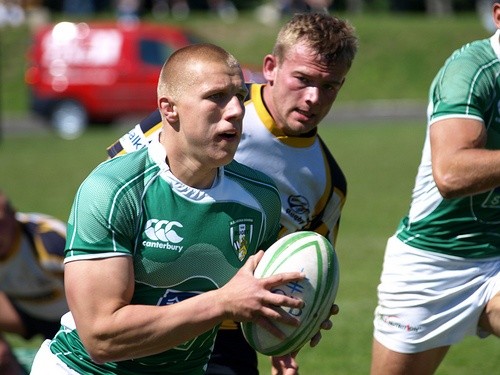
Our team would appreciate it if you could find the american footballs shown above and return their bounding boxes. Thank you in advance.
[238,231,340,356]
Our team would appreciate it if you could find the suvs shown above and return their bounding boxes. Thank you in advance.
[22,20,266,142]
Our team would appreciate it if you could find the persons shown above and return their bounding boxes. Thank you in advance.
[371,0,500,375]
[0,194,70,375]
[31,42,338,375]
[106,12,358,375]
